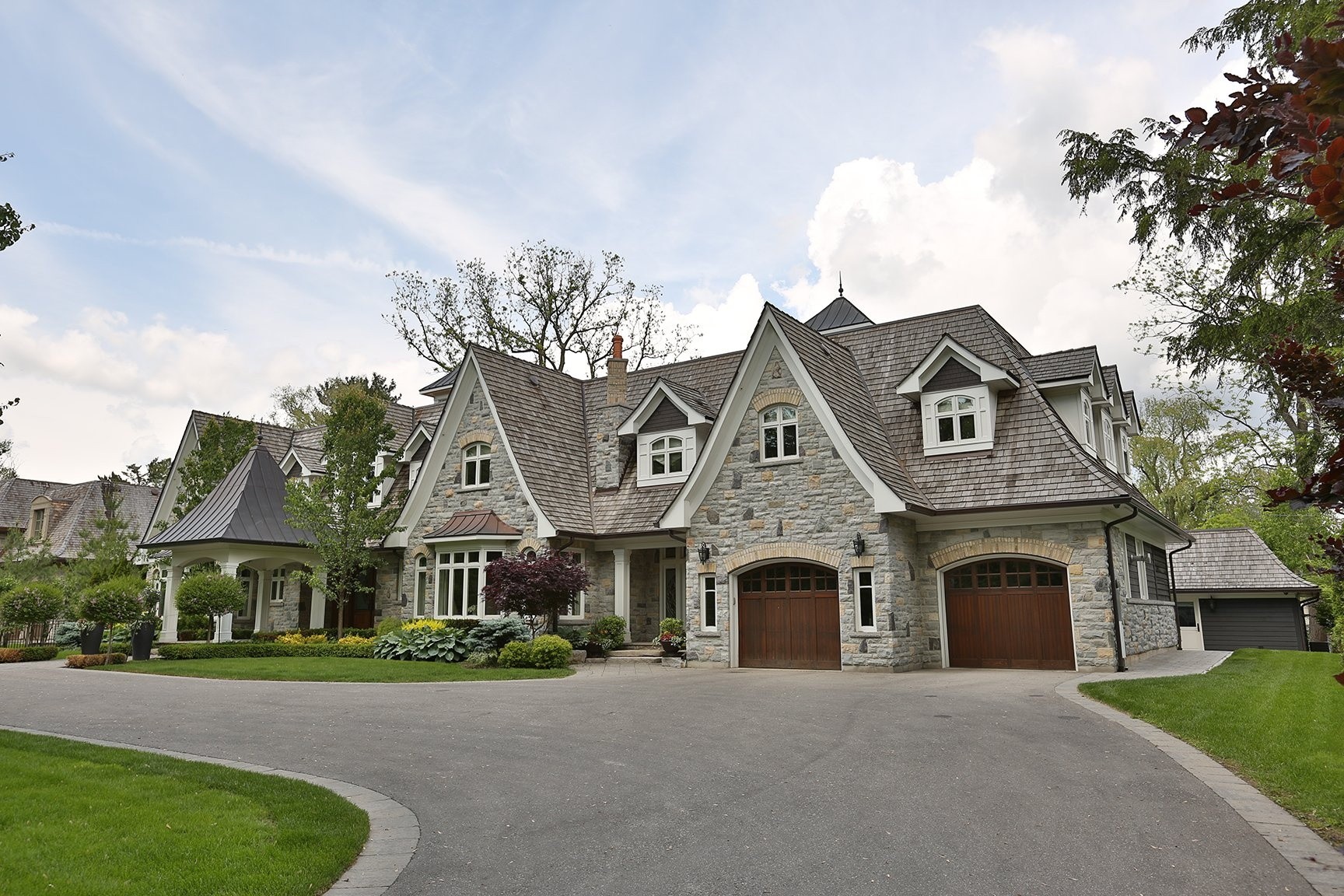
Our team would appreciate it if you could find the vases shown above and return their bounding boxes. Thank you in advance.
[660,640,683,653]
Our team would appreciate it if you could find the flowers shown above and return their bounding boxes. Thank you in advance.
[651,631,686,648]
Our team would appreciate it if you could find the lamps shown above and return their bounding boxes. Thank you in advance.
[1208,595,1215,610]
[698,541,709,565]
[853,532,865,558]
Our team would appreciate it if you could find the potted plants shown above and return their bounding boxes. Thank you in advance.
[579,630,613,654]
[131,590,160,661]
[75,618,104,654]
